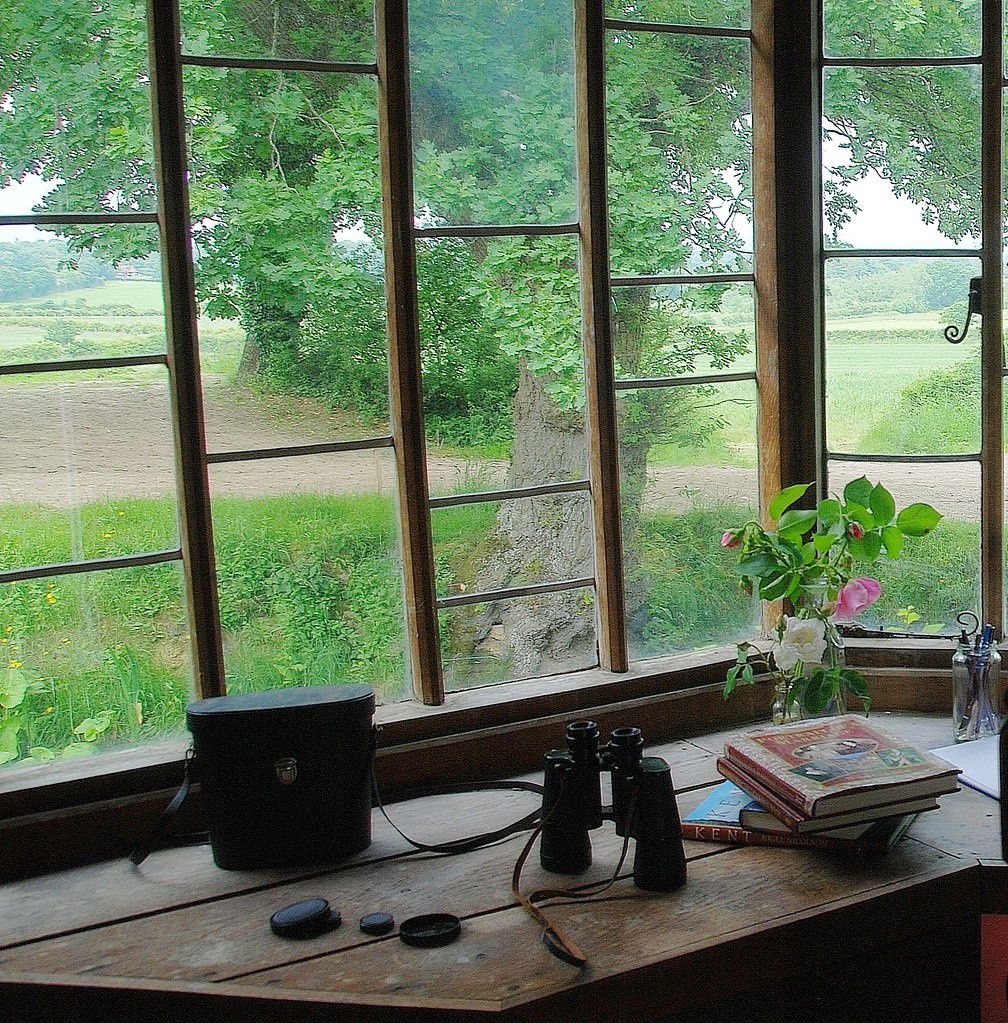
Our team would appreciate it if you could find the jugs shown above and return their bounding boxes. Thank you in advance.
[633,756,687,893]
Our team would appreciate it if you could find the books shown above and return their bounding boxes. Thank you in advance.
[681,713,963,853]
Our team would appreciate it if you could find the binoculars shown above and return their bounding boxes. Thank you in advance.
[540,717,688,892]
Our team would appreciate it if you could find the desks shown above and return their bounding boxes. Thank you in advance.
[0,716,1008,1023]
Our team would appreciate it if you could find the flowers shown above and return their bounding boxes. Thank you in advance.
[717,475,946,718]
[720,612,829,723]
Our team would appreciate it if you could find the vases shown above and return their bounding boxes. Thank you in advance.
[792,575,848,716]
[772,682,801,725]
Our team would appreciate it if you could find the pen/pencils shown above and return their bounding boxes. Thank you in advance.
[958,623,997,738]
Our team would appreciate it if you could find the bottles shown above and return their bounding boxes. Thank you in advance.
[608,727,645,836]
[540,749,592,874]
[952,643,1001,742]
[564,721,603,831]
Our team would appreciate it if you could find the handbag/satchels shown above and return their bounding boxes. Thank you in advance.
[185,684,374,873]
